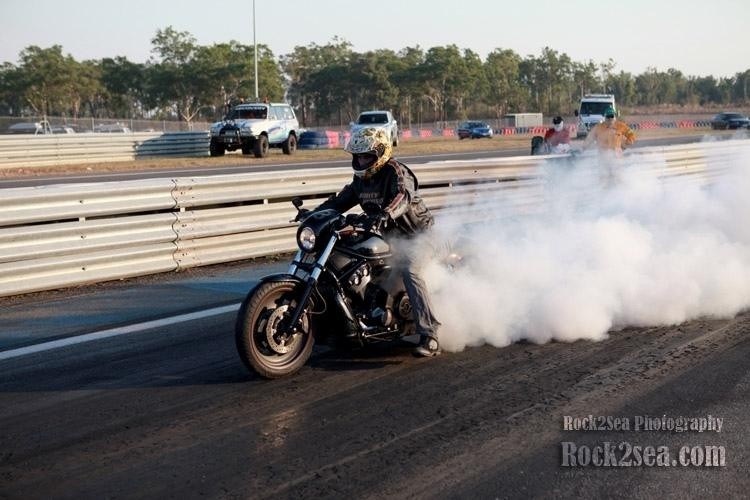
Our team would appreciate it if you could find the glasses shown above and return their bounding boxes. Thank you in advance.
[606,114,616,120]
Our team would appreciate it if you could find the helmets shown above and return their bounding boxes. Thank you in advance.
[343,126,395,179]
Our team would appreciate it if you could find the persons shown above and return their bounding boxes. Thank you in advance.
[294,123,444,358]
[577,106,640,164]
[544,114,570,155]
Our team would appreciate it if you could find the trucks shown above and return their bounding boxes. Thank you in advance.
[576,94,620,139]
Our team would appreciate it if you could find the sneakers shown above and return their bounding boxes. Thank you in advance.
[414,333,443,359]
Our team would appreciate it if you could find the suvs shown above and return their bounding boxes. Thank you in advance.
[210,103,299,157]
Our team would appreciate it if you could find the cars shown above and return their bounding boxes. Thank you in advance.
[349,111,399,146]
[711,112,750,130]
[458,121,493,139]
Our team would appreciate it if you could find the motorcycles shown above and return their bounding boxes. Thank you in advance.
[235,197,464,379]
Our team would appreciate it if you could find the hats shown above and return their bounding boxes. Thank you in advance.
[604,107,617,118]
[552,114,563,122]
